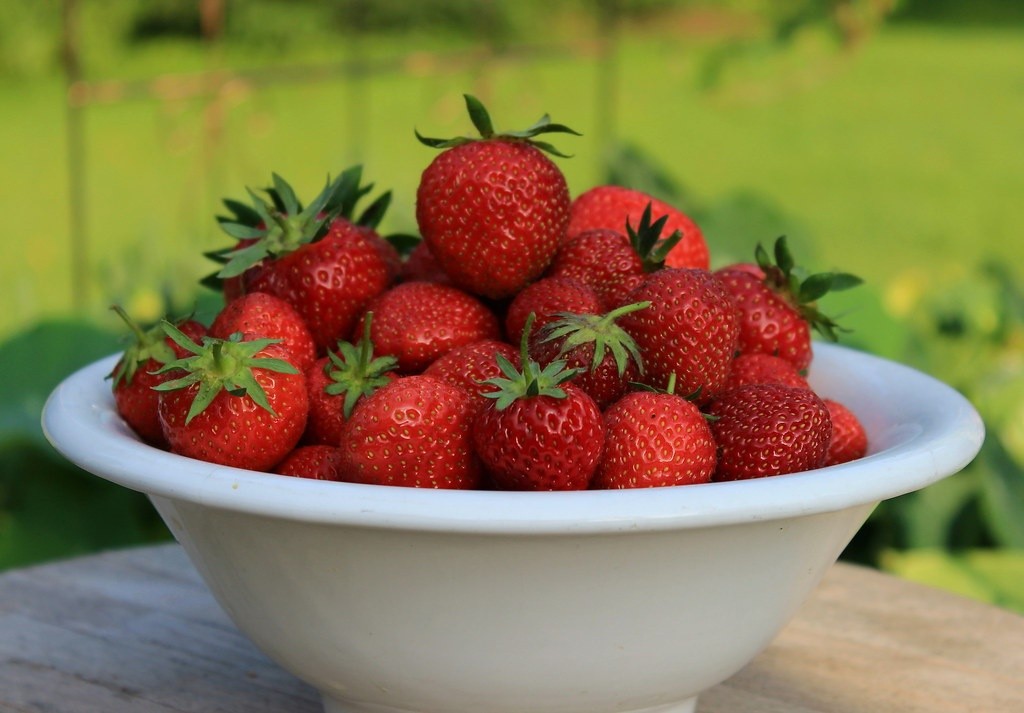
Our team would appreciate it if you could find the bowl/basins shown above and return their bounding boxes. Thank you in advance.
[41,341,985,713]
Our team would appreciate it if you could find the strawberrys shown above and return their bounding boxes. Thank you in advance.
[107,95,866,488]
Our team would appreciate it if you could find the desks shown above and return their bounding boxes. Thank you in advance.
[0,540,1024,713]
